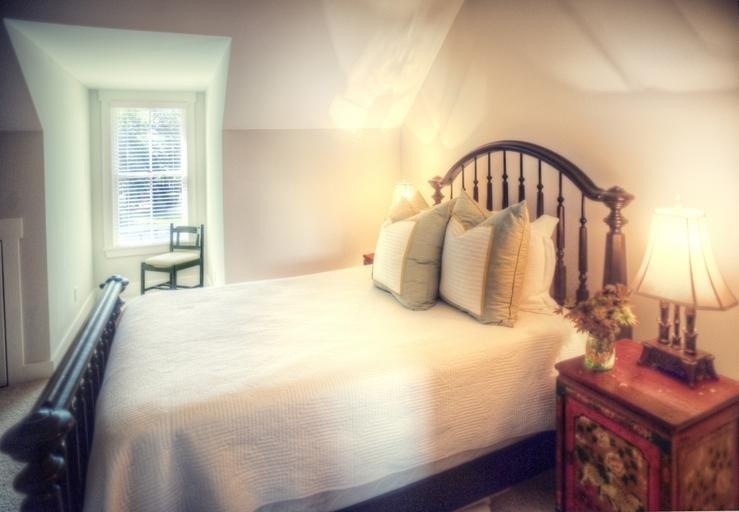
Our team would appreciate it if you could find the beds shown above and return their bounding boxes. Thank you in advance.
[2,141,635,512]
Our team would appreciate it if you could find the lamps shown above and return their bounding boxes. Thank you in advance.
[630,206,739,387]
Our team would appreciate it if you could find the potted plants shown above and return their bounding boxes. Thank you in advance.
[556,283,636,369]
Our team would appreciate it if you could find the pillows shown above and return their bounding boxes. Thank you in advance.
[372,191,560,327]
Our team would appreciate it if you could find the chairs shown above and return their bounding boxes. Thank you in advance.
[141,223,203,294]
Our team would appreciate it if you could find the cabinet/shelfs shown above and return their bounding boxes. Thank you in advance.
[554,339,739,512]
